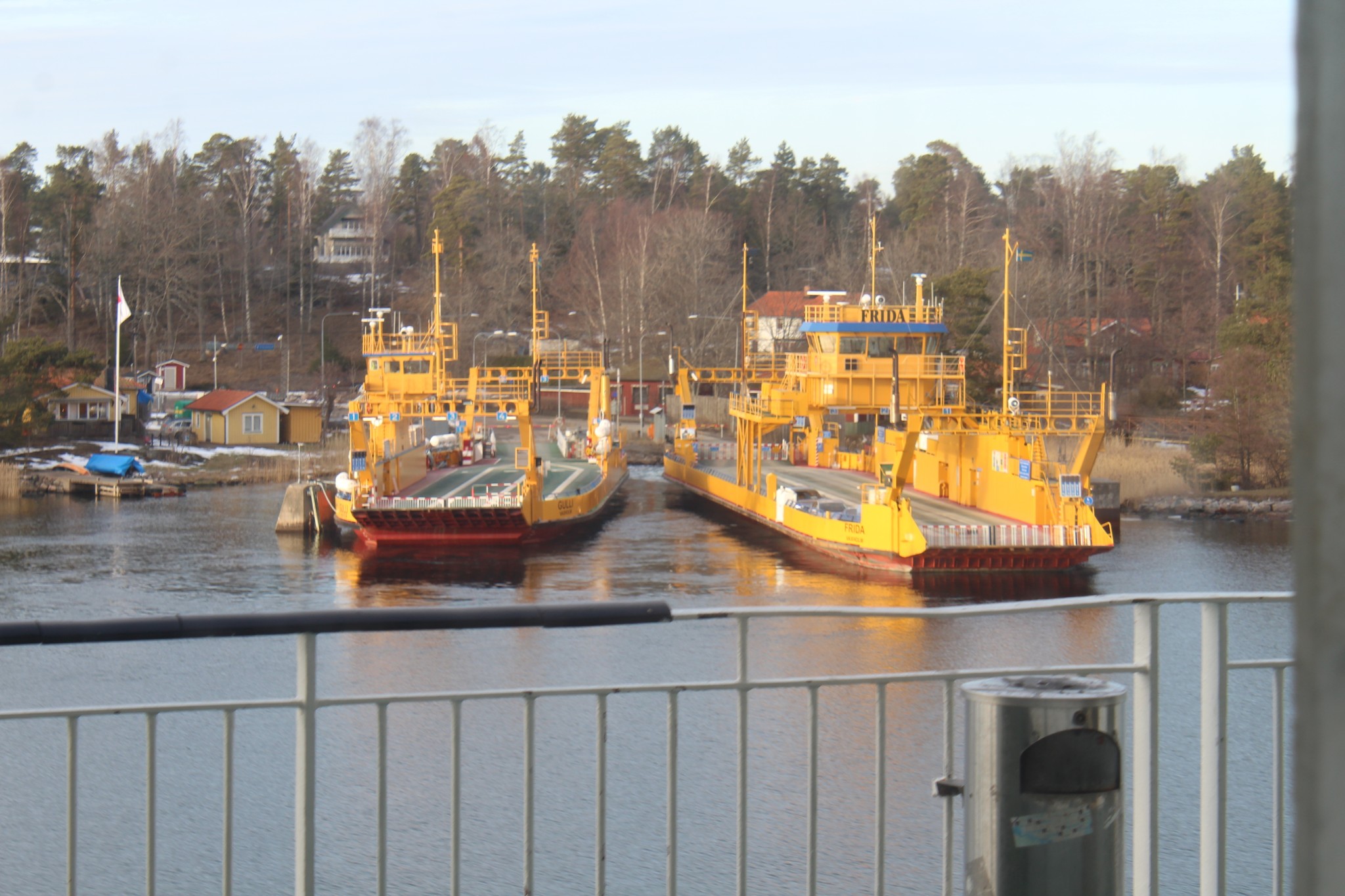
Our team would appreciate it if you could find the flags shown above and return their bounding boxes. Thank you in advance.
[1018,249,1033,261]
[118,277,131,325]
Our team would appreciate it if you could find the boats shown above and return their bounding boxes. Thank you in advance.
[333,228,632,564]
[659,213,1115,574]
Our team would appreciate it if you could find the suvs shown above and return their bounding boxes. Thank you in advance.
[164,419,192,440]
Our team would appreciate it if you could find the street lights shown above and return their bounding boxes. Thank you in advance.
[134,311,151,382]
[531,327,561,417]
[297,442,304,484]
[277,335,283,396]
[322,311,360,400]
[640,331,666,437]
[568,311,593,350]
[688,314,738,435]
[212,334,227,390]
[472,329,517,440]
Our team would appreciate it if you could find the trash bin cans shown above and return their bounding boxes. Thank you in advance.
[961,674,1128,896]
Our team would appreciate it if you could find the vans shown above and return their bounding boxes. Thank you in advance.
[145,412,169,431]
[174,399,194,419]
